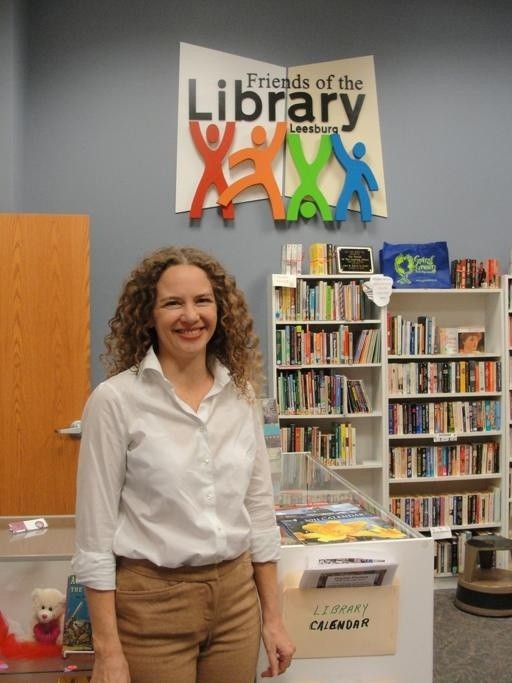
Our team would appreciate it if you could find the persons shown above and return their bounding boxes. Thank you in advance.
[70,245,296,682]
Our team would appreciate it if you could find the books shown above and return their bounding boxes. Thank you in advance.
[299,549,399,587]
[277,326,380,366]
[387,311,486,355]
[387,362,502,395]
[390,485,501,527]
[275,280,366,321]
[281,422,357,467]
[390,444,500,478]
[278,371,372,414]
[388,403,501,434]
[281,243,336,275]
[451,258,500,289]
[434,532,503,574]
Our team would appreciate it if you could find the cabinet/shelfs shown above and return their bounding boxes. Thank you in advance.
[265,450,433,682]
[0,515,94,681]
[384,287,502,593]
[502,275,510,551]
[267,273,385,520]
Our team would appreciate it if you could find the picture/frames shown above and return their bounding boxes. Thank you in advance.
[334,245,374,274]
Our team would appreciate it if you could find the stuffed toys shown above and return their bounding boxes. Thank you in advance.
[30,588,65,645]
